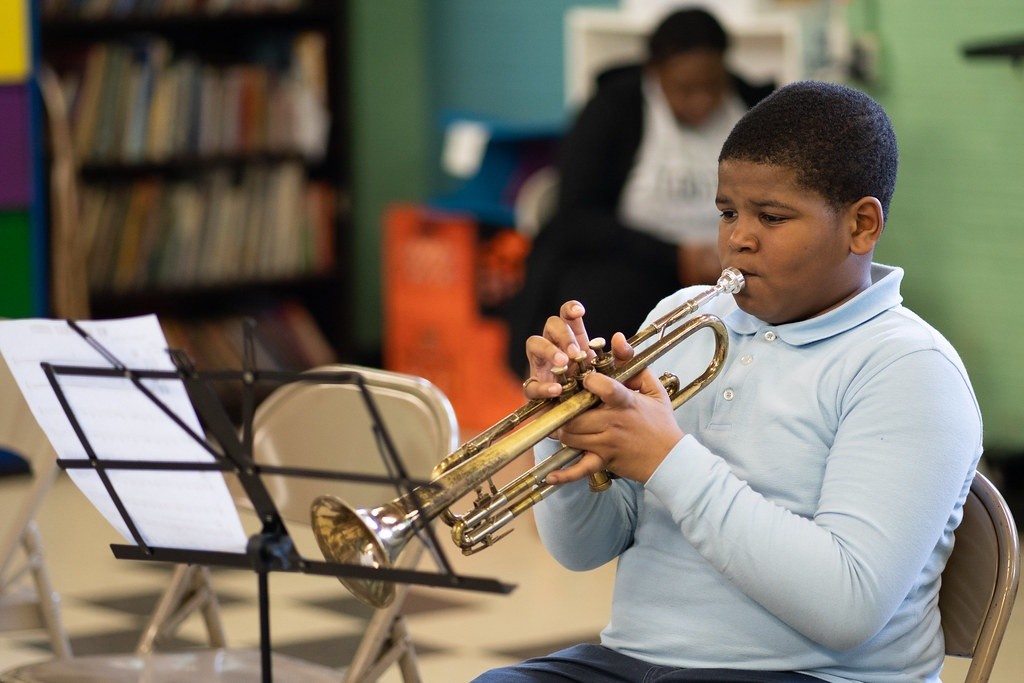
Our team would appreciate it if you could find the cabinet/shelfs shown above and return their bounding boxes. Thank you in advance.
[32,0,361,429]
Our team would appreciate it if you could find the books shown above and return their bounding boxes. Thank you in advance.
[57,35,335,405]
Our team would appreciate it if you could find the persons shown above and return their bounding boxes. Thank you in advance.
[508,9,780,379]
[468,81,983,683]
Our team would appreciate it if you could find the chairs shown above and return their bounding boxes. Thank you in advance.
[0,351,460,683]
[939,471,1024,683]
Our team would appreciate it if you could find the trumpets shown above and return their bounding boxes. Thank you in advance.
[310,264,749,610]
